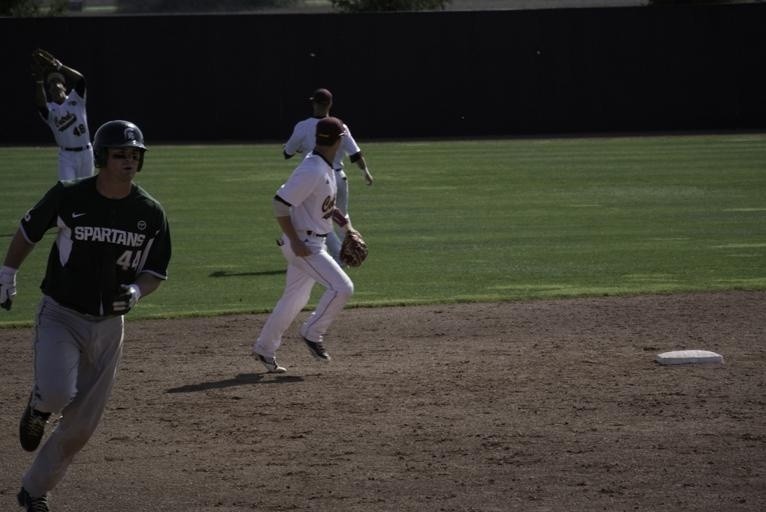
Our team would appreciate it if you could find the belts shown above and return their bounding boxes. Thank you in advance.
[65,144,89,151]
[307,230,328,237]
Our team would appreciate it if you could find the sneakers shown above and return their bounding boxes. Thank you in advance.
[20,391,51,452]
[303,337,330,360]
[17,486,50,512]
[253,353,287,373]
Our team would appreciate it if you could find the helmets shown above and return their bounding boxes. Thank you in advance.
[93,120,149,172]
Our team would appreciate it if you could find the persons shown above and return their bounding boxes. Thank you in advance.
[27,46,97,182]
[0,118,172,511]
[253,116,355,375]
[282,87,374,270]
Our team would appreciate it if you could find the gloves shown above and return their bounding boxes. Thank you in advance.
[112,284,141,311]
[0,266,19,311]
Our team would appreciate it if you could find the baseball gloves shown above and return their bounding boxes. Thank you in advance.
[30,47,61,77]
[339,229,368,269]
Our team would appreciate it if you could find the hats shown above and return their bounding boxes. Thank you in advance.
[317,118,345,138]
[309,88,332,104]
